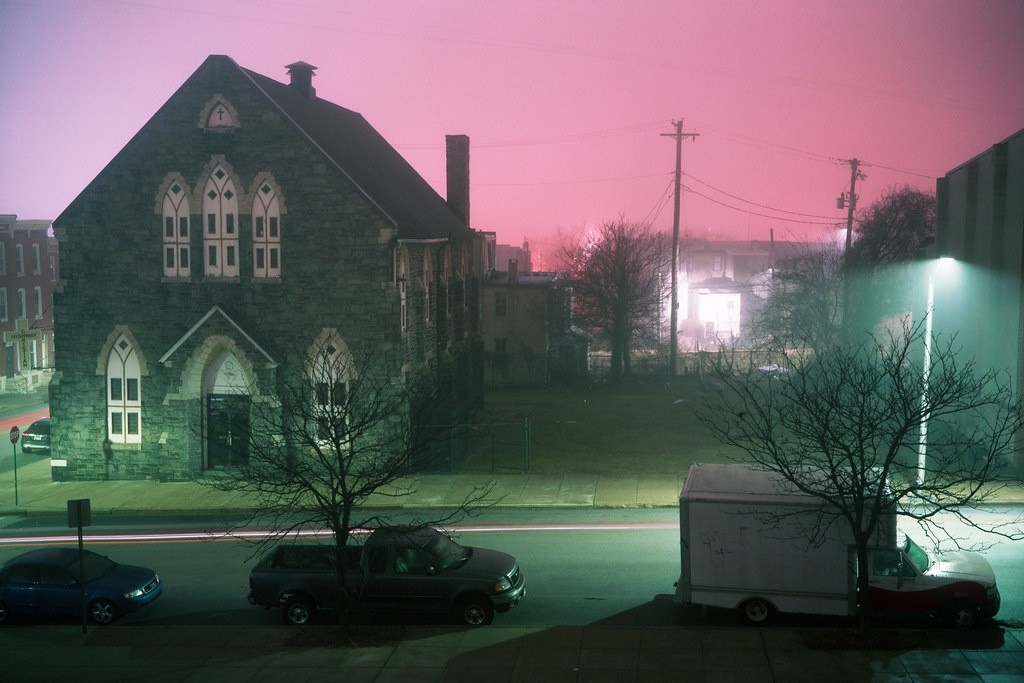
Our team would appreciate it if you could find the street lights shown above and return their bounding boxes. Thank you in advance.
[916,250,956,488]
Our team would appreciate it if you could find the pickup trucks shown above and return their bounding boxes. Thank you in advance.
[247,525,527,625]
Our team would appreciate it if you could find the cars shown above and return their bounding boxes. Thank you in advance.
[0,547,163,627]
[21,418,50,453]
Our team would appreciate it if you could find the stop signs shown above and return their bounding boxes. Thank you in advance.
[9,426,20,444]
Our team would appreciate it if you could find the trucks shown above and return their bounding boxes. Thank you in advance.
[679,462,1002,631]
